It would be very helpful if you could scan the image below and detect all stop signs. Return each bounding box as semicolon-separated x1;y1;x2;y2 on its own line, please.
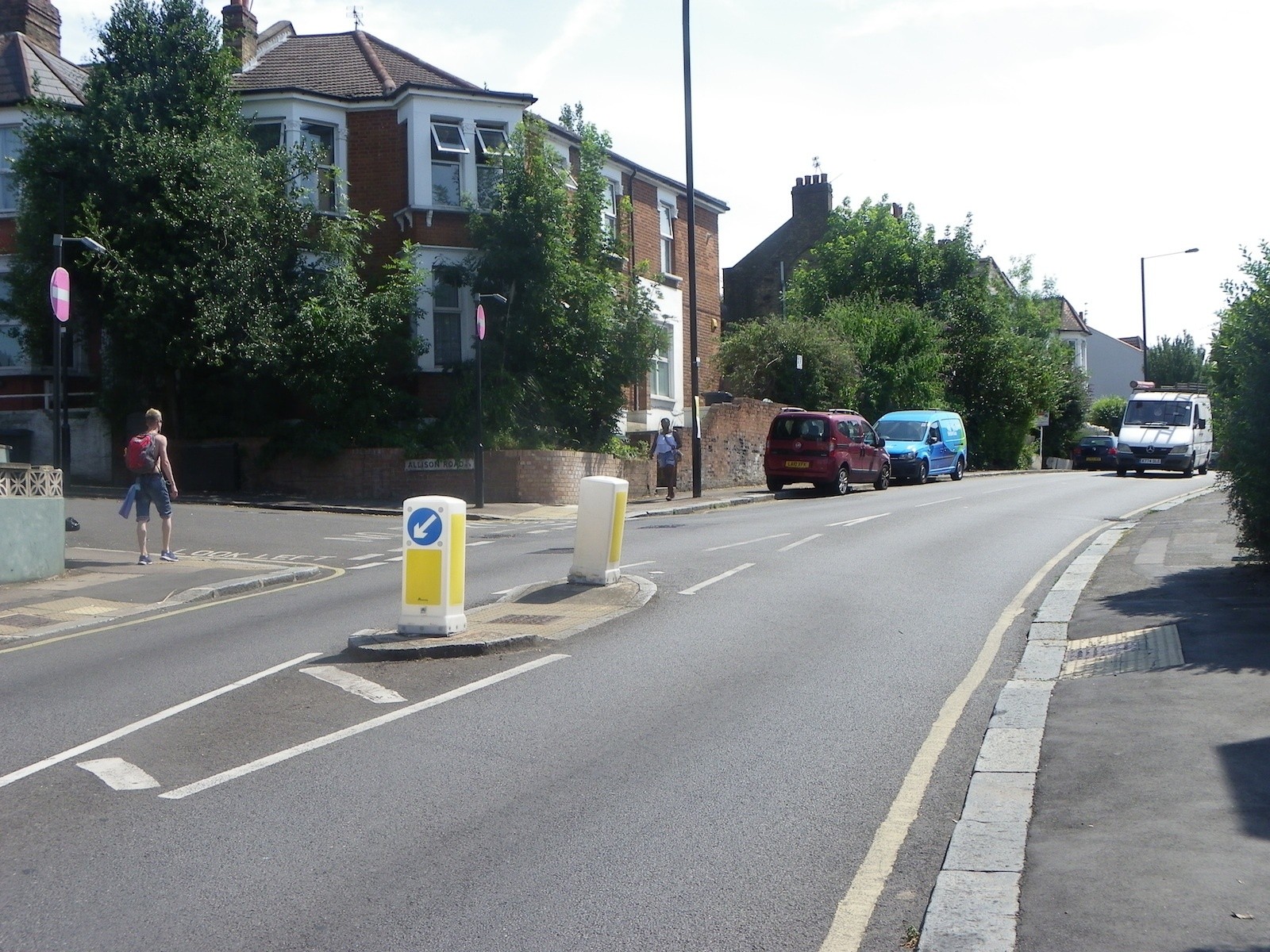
475;305;486;341
51;267;70;323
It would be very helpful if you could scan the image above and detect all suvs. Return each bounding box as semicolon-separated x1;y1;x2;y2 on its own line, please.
764;409;893;496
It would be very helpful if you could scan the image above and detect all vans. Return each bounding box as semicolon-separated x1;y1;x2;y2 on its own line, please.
863;409;968;485
1114;384;1212;478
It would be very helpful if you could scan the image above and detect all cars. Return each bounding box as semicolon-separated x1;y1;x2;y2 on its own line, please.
1074;436;1119;472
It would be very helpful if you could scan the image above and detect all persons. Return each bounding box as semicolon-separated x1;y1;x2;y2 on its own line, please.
132;409;179;565
650;417;683;501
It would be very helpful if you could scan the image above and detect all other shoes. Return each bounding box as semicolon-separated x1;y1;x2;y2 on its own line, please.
675;450;684;459
666;496;672;501
159;550;179;562
138;555;153;565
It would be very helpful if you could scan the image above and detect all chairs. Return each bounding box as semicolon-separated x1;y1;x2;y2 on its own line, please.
807;425;820;441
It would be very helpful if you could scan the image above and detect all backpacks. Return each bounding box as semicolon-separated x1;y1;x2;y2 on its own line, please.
125;433;161;475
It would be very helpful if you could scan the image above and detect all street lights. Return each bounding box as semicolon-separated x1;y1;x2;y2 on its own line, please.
51;233;108;460
1139;248;1200;383
472;291;508;510
